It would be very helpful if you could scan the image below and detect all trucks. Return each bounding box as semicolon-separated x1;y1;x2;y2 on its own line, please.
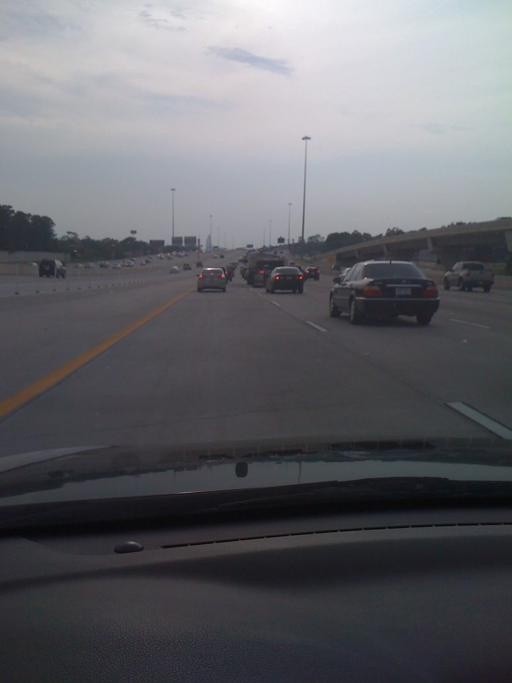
249;254;282;285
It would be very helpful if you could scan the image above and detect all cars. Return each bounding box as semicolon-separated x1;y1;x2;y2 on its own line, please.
39;258;66;279
266;267;304;294
169;260;203;273
196;267;228;292
305;266;320;280
443;261;495;292
329;260;440;326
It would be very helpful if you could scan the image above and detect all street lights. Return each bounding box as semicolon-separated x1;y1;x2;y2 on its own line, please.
170;188;176;246
301;135;311;240
268;217;272;247
288;201;294;249
